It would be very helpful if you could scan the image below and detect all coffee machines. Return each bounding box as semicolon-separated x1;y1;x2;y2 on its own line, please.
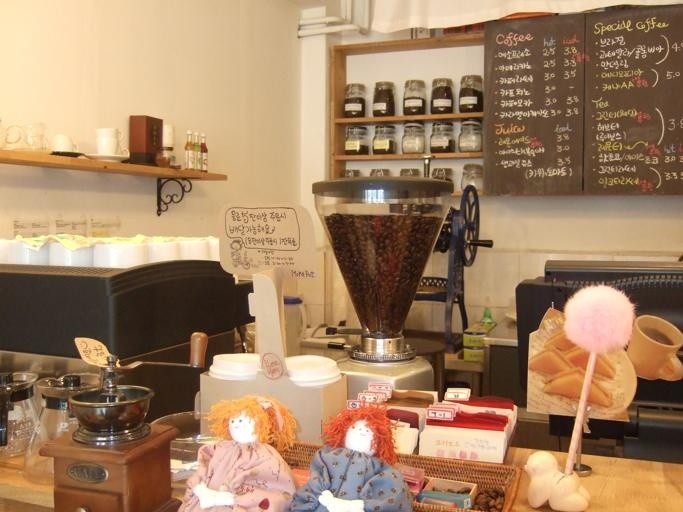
312;177;454;400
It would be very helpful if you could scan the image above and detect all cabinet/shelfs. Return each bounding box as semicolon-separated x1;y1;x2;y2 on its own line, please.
331;31;483;196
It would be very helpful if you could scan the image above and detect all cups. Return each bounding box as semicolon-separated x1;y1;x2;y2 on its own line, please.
627;315;683;382
96;128;125;155
284;355;341;388
209;353;261;380
52;134;79;153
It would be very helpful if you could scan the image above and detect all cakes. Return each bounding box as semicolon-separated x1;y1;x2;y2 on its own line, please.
528;330;615;407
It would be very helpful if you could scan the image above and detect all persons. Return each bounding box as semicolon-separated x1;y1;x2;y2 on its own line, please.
176;393;297;512
290;407;414;512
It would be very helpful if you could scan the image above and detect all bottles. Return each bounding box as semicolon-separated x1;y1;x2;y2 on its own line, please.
184;131;208;172
25;371;100;477
343;75;483;118
341;164;484;192
0;370;42;458
344;118;482;155
154;146;175;166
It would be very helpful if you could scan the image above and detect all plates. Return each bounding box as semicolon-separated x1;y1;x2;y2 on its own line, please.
527;324;638;421
49;151;85;158
84;154;129;163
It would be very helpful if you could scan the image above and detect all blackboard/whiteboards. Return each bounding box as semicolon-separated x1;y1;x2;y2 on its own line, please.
583;4;683;196
482;13;585;196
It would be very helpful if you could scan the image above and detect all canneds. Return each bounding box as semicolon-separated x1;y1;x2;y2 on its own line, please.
430;122;455;152
458;119;482;152
401;122;425;154
373;126;395;154
344;127;368;155
